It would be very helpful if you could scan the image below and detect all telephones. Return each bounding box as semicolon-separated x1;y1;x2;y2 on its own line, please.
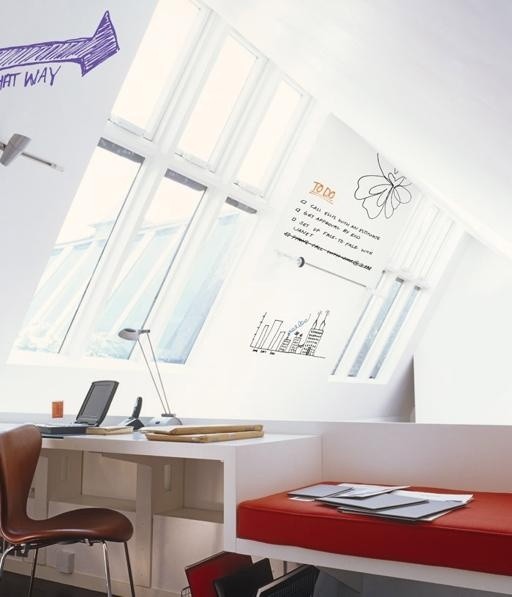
120;397;145;429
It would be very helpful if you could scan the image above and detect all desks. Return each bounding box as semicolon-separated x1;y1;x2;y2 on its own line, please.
237;479;510;596
1;422;323;596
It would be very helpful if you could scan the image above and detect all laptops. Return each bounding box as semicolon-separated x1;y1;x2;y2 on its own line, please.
33;380;118;435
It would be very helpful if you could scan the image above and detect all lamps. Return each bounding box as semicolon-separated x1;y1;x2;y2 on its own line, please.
118;328;182;428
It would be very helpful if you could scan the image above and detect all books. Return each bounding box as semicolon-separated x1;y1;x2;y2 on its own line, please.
286;478;477;525
85;425;134;435
212;558;273;597
184;551;253;597
254;564;320;597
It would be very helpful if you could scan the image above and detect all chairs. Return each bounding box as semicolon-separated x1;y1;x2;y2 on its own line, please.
1;424;134;597
